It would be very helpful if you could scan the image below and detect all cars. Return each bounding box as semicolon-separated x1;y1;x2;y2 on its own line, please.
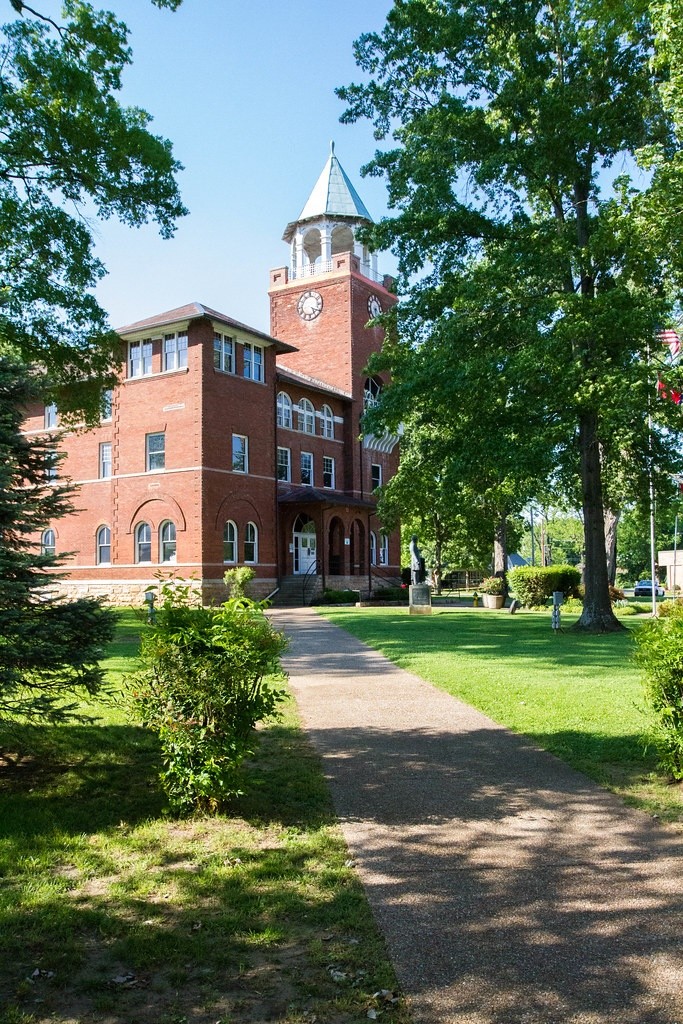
634;580;664;597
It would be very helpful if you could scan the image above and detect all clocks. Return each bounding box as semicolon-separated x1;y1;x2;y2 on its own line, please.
367;294;382;319
296;290;323;321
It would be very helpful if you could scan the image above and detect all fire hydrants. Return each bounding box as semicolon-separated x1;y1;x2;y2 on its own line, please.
472;591;478;607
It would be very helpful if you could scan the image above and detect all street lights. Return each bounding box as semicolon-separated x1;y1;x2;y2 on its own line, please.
530;506;539;566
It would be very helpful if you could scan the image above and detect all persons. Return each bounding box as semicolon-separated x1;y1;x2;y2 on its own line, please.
408;535;422;584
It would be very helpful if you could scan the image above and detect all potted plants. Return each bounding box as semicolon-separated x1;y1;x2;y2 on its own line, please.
478;576;508;609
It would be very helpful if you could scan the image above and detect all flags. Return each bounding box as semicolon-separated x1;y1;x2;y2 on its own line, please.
650;326;682;404
